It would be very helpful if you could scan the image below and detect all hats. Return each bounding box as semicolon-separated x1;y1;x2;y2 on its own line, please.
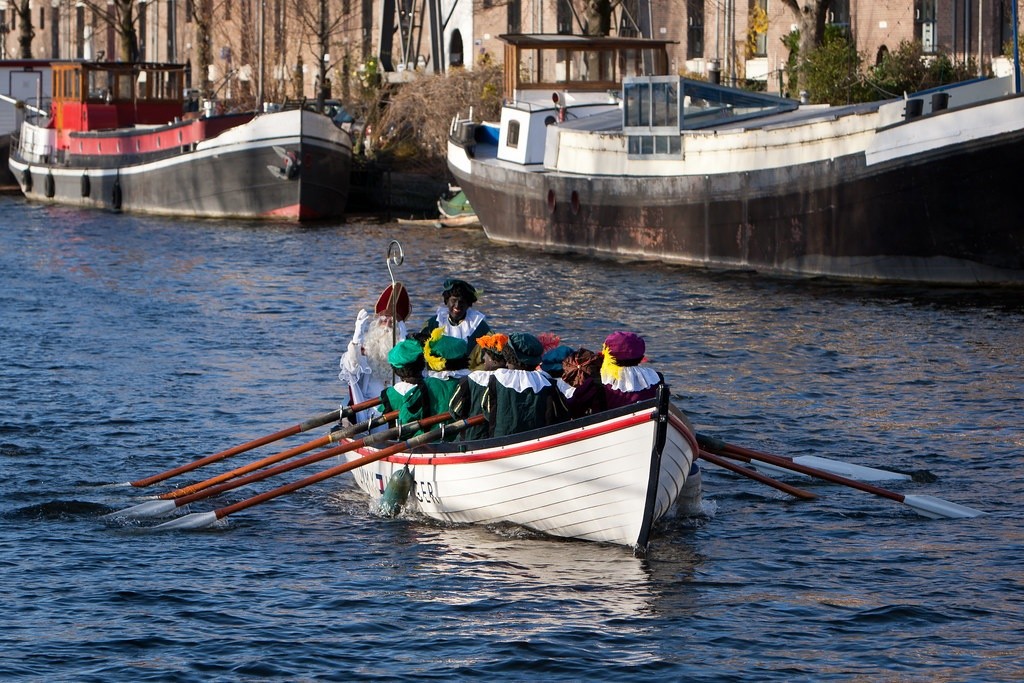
476;332;544;366
442;279;484;304
387;340;423;369
602;331;647;379
538;331;604;386
423;327;467;372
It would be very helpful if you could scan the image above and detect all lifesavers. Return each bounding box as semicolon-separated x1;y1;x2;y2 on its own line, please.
44;174;55;196
20;169;32;192
80;175;90;197
111;186;122;209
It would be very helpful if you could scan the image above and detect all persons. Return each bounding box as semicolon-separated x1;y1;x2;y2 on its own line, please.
340;278;664;443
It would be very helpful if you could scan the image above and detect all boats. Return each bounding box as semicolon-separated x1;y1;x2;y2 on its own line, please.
8;55;354;227
329;372;703;558
441;0;1024;292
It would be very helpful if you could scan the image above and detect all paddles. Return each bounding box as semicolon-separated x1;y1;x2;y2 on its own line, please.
101;394;487;534
695;431;987;520
